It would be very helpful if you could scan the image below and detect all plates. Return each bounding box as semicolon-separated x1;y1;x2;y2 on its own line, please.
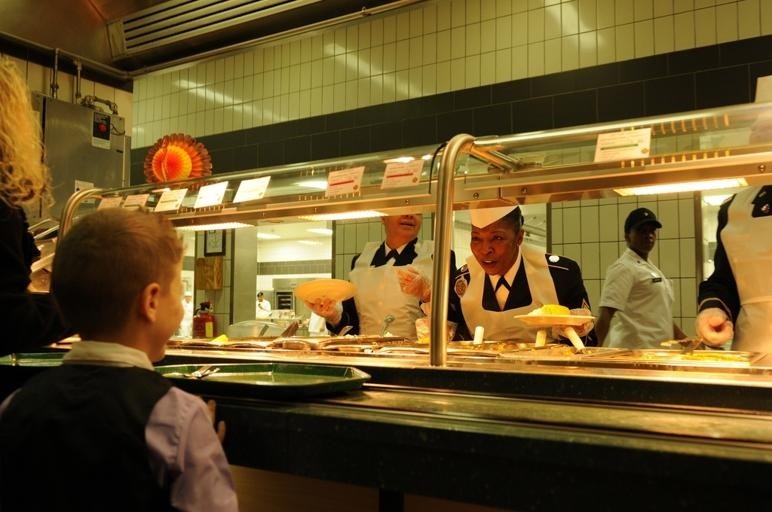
293;279;359;303
515;315;596;328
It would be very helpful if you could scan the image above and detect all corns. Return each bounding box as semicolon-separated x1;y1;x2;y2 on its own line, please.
541;305;570;316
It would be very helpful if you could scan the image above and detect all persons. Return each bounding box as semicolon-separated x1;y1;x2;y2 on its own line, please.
451;205;598;346
0;207;239;512
696;175;772;351
304;214;457;342
181;291;193;327
595;208;691;349
256;292;271;317
0;57;78;357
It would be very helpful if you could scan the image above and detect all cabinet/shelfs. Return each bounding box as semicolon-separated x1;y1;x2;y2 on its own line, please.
57;98;772;512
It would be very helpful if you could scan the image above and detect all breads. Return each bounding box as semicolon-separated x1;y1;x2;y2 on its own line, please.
570;307;591;316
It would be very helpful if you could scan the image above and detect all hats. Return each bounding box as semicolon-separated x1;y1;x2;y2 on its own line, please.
471;206;518;229
755;74;772;104
625;208;662;228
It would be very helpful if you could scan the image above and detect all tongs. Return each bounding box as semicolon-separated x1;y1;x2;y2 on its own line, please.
187;366;221;382
271;320;299;337
